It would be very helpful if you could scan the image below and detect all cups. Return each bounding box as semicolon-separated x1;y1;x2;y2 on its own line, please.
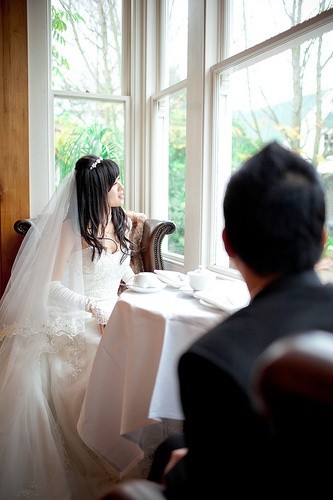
128;272;156;287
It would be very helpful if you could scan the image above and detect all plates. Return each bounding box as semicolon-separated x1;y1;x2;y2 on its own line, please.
179;285;195;296
199;299;223;310
126;282;167;293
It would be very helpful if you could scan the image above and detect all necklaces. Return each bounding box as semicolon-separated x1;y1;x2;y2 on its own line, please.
98;213;112;225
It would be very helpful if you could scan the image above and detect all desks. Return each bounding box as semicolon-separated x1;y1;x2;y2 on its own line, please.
77;286;251;477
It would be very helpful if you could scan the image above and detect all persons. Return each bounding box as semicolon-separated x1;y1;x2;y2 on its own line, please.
145;141;333;500
0;154;137;500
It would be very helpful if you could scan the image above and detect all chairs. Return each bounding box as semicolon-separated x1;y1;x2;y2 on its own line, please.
13;212;176;295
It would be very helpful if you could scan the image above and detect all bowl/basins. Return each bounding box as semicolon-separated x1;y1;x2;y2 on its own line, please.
187;266;218;290
